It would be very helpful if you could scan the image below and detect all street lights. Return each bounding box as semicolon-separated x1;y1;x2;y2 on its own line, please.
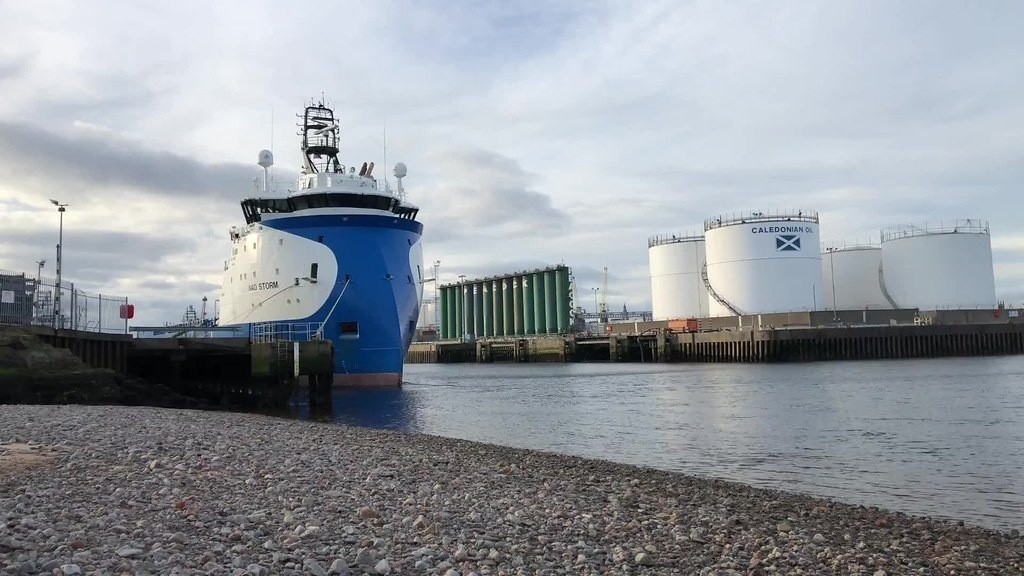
459;274;467;343
49;197;70;329
35;259;46;326
591;288;600;337
826;247;840;326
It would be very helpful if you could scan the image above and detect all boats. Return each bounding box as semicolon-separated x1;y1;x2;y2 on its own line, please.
137;91;435;390
